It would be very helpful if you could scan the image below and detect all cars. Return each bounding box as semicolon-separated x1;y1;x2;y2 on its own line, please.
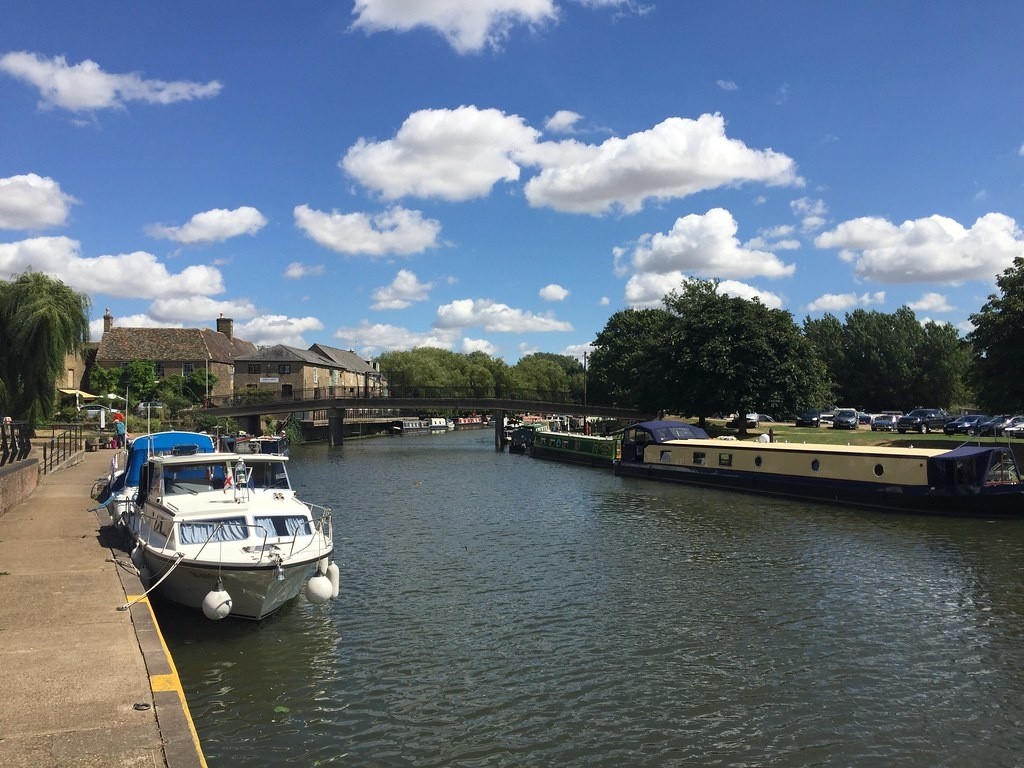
795;411;821;427
943;414;991;436
833;407;861;430
979;415;1024;437
1001;416;1024;438
55;404;120;421
734;410;760;428
133;401;167;416
870;415;898;432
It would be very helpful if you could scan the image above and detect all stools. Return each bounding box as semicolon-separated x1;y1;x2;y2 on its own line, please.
90;442;105;451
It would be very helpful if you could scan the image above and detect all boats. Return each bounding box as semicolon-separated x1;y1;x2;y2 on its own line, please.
508;414;570;454
534;431;622;468
101;387;334;622
615;420;1024;520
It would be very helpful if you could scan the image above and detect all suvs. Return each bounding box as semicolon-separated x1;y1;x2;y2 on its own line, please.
897;409;955;435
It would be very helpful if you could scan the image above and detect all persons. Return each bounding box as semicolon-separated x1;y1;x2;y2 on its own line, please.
114;419;126;448
